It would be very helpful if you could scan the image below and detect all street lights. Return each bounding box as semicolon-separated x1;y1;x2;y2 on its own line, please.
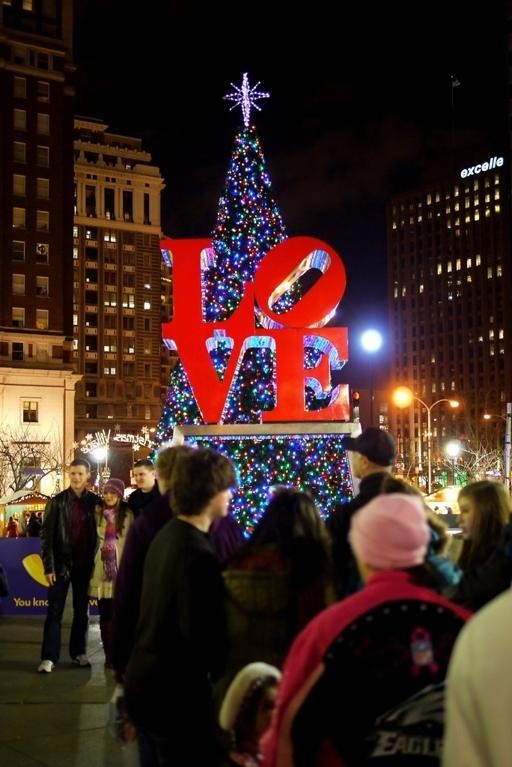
483;410;511;496
394;386;461;497
446;441;482;482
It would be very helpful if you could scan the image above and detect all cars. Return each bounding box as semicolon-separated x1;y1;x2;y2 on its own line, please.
422;484;463;531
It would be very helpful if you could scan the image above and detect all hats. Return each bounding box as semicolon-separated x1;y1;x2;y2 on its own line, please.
105;479;125;496
341;427;397;466
348;493;429;568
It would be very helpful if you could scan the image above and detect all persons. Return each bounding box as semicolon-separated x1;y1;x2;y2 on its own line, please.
104;423;511;767
84;477;135;669
34;457;105;674
6;510;43;537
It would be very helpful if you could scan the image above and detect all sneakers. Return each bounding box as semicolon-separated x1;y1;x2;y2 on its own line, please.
74;655;92;668
39;660;54;673
